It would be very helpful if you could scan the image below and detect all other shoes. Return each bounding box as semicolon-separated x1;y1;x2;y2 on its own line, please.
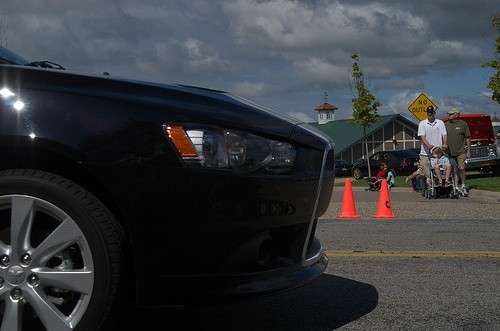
445;180;451;184
438;181;442;184
461;187;469;197
454;188;460;196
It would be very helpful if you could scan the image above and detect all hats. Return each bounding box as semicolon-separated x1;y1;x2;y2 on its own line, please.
447;109;460;115
380;162;385;167
426;106;434;112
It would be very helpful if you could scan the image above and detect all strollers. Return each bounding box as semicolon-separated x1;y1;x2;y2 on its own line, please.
424;145;460;200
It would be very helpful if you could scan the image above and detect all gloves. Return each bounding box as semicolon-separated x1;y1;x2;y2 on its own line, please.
405;177;409;183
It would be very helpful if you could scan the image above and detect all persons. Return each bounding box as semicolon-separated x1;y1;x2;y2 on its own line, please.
405;158;423;192
365;162;396;191
417;105;447;177
443;108;471;197
430;147;451;184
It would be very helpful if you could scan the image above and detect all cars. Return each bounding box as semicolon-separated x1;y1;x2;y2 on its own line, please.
352;149;421;180
403;148;422;155
335;159;352;177
0;43;336;331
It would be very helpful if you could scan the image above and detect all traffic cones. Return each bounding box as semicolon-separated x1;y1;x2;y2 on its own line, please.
372;178;397;218
336;179;362;218
